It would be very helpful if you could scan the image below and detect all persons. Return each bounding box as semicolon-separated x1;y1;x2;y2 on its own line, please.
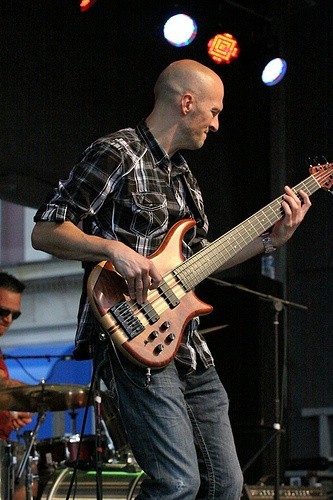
31;59;313;500
0;270;97;500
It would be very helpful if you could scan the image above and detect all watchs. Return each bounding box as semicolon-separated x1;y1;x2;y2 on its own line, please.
262;232;276;254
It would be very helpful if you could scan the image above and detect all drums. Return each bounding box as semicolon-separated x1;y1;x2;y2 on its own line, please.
45;432;115;469
35;434;69;467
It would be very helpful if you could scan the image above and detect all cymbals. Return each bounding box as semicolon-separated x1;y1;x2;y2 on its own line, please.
1;384;94;410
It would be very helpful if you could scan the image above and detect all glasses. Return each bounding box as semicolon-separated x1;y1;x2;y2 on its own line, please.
0;305;21;320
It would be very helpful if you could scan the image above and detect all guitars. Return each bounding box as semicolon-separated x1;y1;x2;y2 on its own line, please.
88;163;333;368
